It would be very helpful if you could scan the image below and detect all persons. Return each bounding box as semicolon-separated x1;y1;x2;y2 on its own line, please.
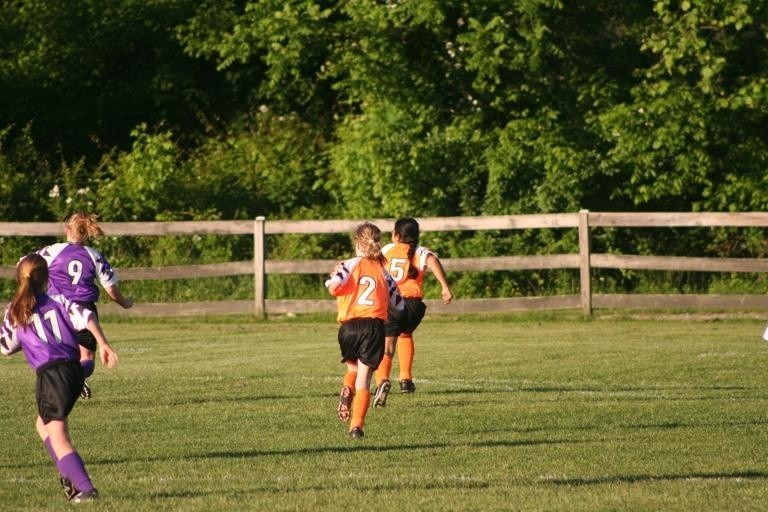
16;212;136;399
324;220;407;440
0;253;120;504
371;218;454;411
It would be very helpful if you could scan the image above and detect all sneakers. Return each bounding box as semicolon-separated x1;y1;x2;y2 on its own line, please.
337;385;353;423
372;379;391;408
400;379;415;392
80;381;91;400
60;477;98;503
349;427;364;441
